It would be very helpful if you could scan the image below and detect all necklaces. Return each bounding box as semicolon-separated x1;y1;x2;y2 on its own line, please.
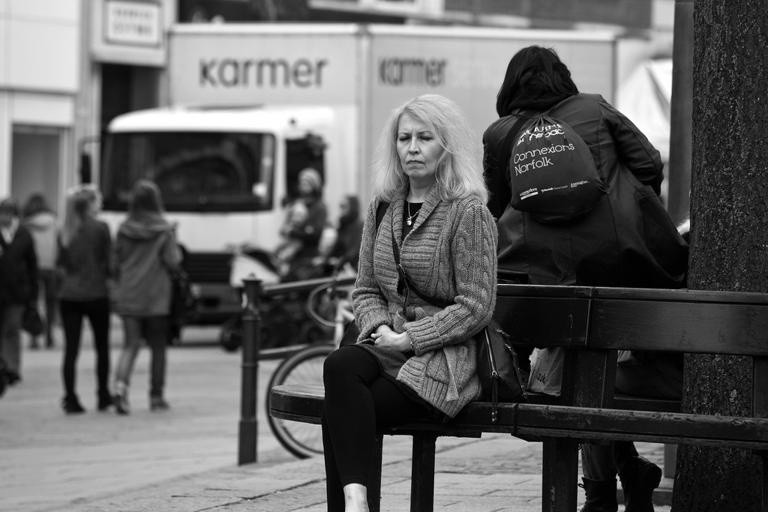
406;201;419;227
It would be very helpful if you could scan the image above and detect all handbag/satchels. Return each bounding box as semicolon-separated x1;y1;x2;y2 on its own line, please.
478;320;524;402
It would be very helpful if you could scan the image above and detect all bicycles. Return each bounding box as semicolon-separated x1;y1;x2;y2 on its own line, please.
267;255;358;458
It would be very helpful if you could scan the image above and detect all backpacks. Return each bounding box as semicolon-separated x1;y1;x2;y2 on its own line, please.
508;111;604;215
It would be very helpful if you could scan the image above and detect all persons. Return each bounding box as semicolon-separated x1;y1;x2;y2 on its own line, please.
577;219;691;512
322;93;527;512
107;179;178;414
54;183;115;413
319;195;365;279
281;168;327;282
0;198;39;386
483;46;690;391
21;195;63;350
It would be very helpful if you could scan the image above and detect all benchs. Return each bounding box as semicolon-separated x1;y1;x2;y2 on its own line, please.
267;282;768;511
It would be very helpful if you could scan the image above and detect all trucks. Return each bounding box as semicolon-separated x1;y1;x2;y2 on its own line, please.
76;25;620;321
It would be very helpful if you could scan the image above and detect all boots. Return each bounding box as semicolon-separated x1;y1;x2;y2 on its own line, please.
579;457;662;512
